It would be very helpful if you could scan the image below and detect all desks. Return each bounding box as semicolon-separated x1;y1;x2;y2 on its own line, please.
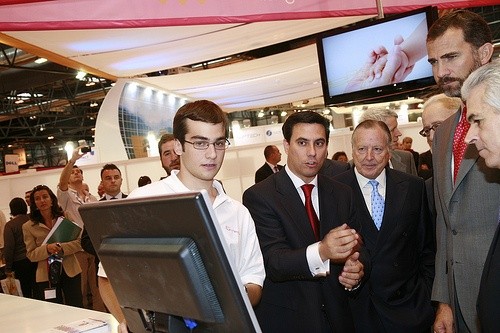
0;288;121;333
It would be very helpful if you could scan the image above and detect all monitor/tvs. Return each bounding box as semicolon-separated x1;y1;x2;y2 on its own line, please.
316;6;438;108
76;192;263;333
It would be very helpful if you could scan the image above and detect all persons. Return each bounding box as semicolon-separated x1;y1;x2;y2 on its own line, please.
0;146;129;314
138;176;151;187
344;17;429;93
241;93;461;333
425;10;500;333
96;100;266;333
158;134;181;177
460;60;499;333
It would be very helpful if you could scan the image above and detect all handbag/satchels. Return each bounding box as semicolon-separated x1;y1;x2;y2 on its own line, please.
47;257;62;289
1;275;24;297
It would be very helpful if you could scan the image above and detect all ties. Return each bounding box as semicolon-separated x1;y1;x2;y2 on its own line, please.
370;181;384;231
301;183;320;242
275;167;278;172
453;106;470;185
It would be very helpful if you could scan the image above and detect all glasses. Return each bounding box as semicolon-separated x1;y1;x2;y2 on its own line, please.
419;123;441;137
184;138;230;150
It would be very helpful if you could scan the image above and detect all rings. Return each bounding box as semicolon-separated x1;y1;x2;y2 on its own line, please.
355;279;359;284
56;243;61;247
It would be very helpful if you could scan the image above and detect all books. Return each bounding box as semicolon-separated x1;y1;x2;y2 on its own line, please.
41;216;83;246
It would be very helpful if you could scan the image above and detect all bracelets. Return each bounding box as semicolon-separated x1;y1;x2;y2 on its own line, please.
68;160;75;165
